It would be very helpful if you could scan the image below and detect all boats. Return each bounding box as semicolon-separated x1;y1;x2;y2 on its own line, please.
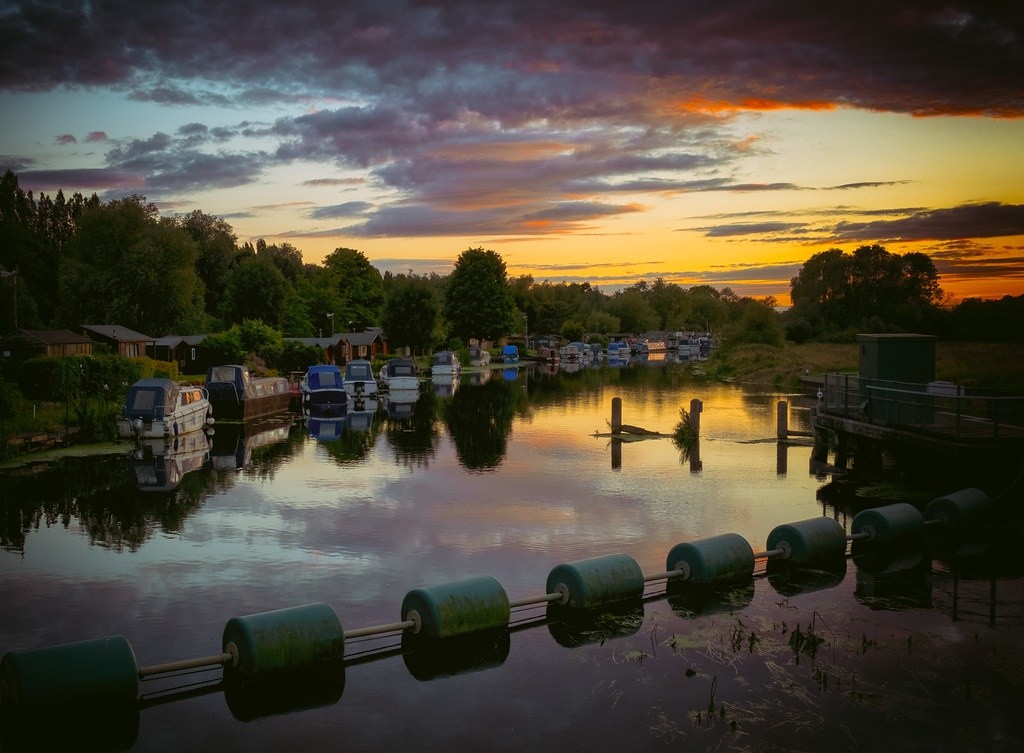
380;359;421;390
301;364;353;405
536;338;709;360
204;365;292;423
430;351;461;375
470;347;492;366
344;360;378;398
501;346;520;363
115;378;211;439
